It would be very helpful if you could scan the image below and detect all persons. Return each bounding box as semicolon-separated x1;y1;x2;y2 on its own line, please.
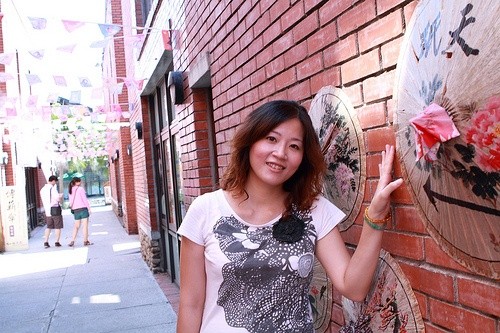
40;175;63;248
68;177;91;247
176;100;403;333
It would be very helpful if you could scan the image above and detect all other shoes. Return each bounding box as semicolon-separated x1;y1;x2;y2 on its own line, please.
69;241;74;247
84;241;93;246
44;242;50;247
55;241;61;246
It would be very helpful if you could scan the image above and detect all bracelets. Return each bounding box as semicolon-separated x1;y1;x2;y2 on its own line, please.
363;215;384;230
365;207;392;223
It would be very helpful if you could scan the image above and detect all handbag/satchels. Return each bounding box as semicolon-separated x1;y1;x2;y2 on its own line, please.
51;207;61;216
